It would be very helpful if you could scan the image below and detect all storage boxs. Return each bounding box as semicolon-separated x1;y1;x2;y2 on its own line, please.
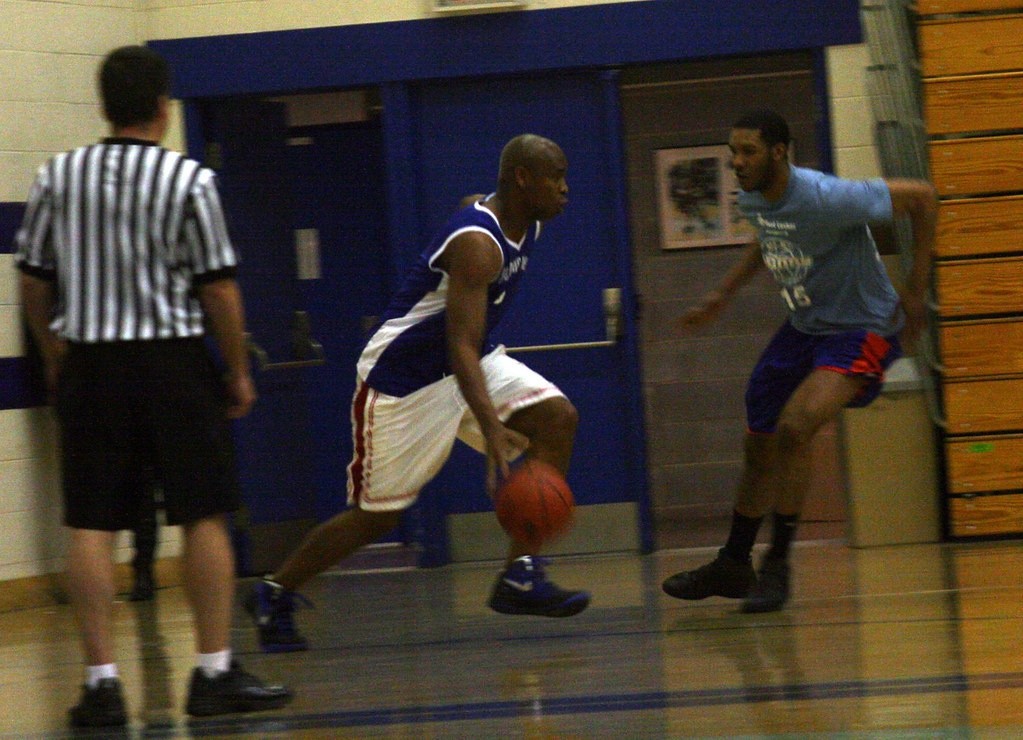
909;0;1023;539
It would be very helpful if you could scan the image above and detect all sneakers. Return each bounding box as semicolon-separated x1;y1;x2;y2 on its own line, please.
187;660;294;716
67;679;127;726
662;556;752;600
490;554;589;617
240;575;315;652
742;562;788;611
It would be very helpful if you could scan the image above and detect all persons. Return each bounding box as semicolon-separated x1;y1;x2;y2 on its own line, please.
11;44;294;731
241;134;591;654
659;111;940;615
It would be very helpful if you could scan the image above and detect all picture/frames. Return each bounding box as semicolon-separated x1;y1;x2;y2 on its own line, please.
651;138;797;254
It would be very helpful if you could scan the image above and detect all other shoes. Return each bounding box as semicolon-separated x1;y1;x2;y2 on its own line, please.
129;585;156;601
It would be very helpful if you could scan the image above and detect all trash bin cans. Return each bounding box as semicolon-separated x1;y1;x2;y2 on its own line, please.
840;357;947;548
797;417;849;522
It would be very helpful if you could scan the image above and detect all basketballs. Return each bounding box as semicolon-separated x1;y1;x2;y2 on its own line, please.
495;458;577;547
